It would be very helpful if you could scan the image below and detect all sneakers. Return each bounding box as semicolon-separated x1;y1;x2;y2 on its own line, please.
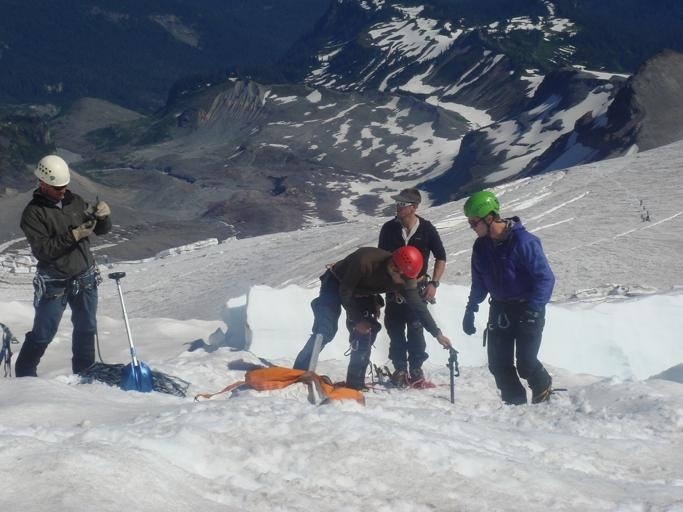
533;377;553;404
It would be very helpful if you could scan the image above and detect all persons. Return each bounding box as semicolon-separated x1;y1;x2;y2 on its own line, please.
15;154;113;378
291;187;453;392
462;190;556;407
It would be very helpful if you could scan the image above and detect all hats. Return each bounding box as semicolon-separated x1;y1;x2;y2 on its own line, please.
391;187;421;204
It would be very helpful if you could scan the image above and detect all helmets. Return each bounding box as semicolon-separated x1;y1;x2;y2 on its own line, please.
34;155;70;187
464;191;501;217
393;245;424;280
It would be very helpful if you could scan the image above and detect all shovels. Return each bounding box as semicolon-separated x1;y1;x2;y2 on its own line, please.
108;272;154;390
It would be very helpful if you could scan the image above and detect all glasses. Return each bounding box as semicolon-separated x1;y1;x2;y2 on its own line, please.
395;201;411;207
470;219;483;228
